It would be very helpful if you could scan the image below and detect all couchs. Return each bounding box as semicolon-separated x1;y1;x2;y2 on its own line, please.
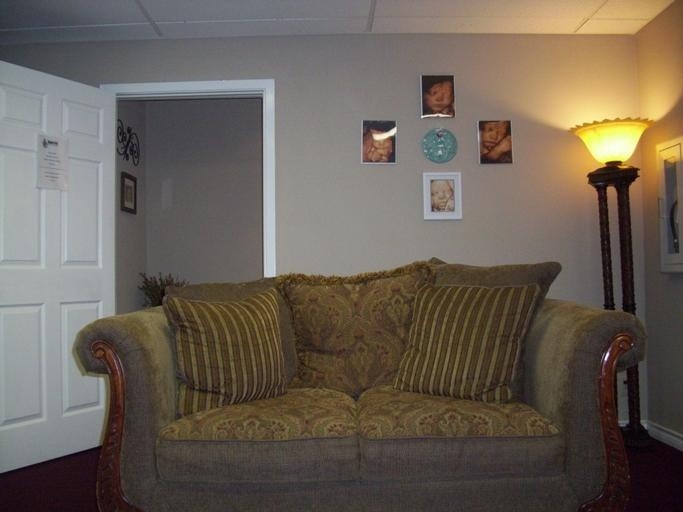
70;295;649;512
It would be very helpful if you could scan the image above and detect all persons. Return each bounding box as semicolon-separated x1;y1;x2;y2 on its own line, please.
363;128;393;164
429;180;454;213
422;79;454;117
478;120;512;165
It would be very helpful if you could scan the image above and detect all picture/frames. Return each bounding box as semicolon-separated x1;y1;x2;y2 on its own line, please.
120;171;137;214
423;172;462;222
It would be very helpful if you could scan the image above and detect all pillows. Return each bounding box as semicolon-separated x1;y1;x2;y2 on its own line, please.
393;277;540;401
162;288;286;421
278;261;434;397
165;276;299;388
427;258;562;297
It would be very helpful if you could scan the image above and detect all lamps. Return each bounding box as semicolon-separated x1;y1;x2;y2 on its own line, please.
572;118;653;448
